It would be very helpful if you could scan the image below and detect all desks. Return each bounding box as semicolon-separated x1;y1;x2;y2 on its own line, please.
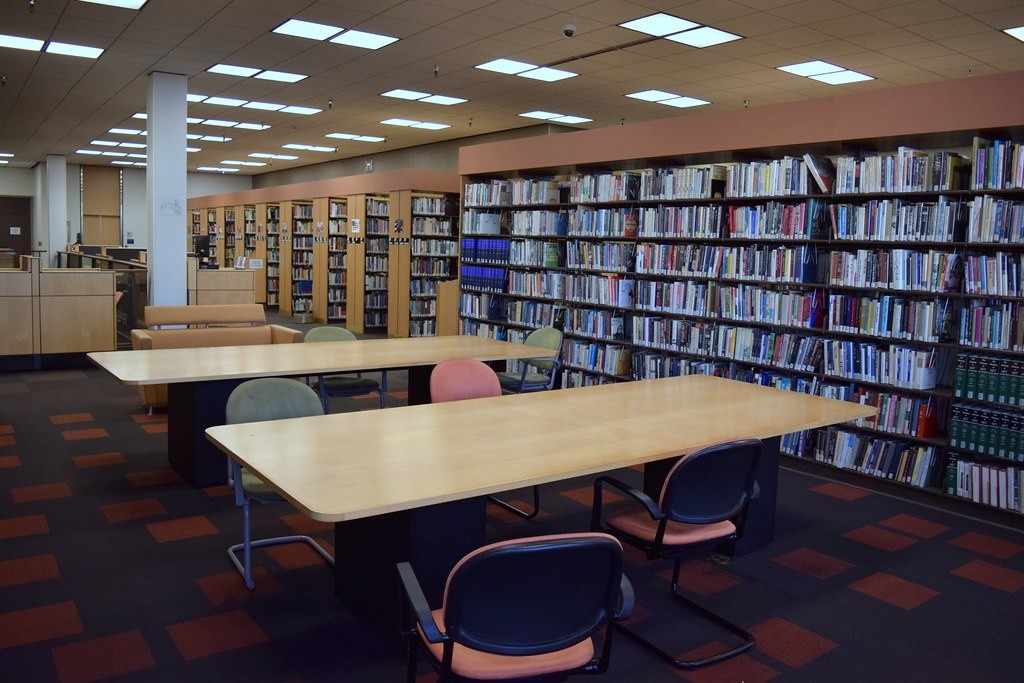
203;372;878;636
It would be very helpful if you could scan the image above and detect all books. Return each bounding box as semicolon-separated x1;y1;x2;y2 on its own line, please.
193;196;458;337
459;137;1024;515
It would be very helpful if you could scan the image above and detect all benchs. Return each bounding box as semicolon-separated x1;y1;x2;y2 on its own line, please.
128;303;305;417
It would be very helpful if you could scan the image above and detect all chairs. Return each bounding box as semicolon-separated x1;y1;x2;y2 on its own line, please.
394;532;624;683
429;358;541;519
86;333;558;490
582;436;767;668
495;327;563;397
225;378;335;585
303;325;388;416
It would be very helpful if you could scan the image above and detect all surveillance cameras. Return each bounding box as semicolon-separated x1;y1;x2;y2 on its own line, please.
561;24;576;38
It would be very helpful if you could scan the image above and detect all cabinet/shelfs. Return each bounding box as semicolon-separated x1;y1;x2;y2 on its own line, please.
184;70;1024;514
0;256;118;368
